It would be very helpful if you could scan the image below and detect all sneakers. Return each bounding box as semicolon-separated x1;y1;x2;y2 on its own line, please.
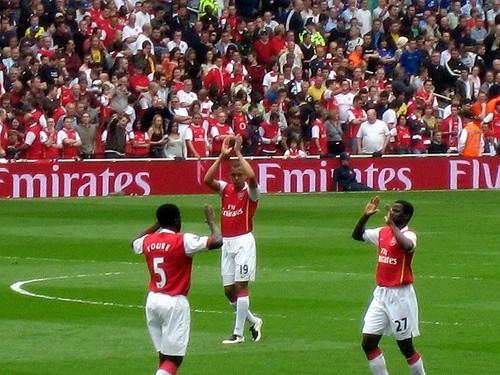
221;335;245;344
249;318;263;342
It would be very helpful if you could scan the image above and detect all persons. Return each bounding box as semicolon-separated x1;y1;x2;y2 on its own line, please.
0;0;500;193
352;196;427;375
204;134;263;344
130;203;223;375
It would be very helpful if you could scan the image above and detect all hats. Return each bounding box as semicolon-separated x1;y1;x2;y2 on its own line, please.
339;151;351;161
